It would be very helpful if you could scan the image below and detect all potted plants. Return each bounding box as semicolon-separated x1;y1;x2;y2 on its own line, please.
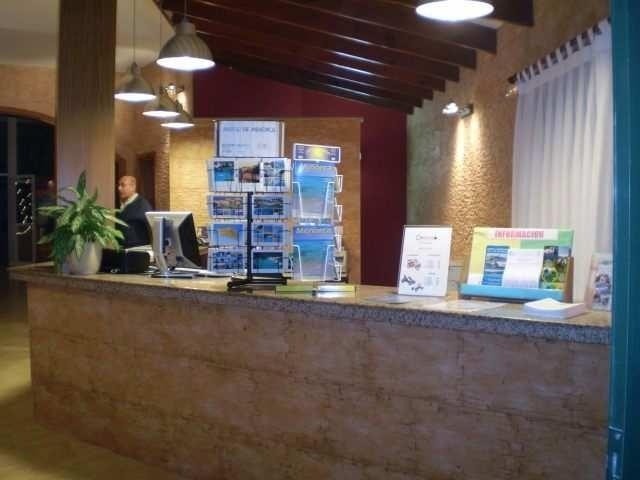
34;166;132;276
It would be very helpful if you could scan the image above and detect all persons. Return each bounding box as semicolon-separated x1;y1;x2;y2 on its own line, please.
116;175;153;249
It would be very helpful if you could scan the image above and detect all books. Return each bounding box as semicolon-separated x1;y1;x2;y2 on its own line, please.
208;158;337;281
523;297;589;320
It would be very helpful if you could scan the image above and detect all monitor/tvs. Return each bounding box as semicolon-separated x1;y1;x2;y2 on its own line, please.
144;211;203;278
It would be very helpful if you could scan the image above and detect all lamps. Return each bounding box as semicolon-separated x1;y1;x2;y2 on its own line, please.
113;1;215;129
